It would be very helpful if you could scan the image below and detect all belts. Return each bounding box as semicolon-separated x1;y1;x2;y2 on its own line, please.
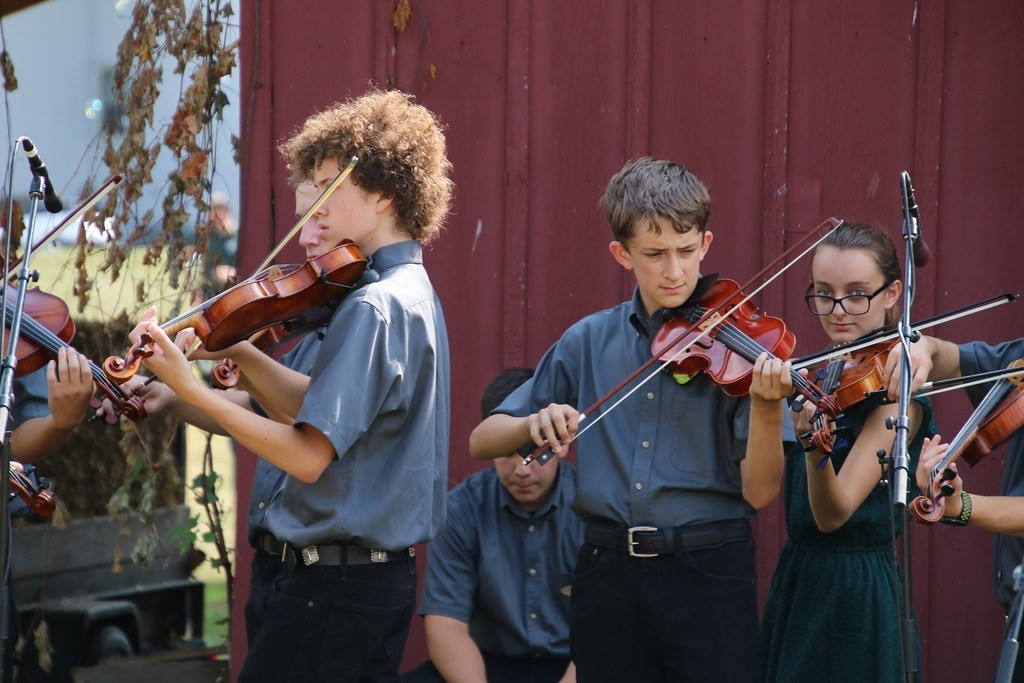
261;532;284;555
281;542;415;571
586;519;752;557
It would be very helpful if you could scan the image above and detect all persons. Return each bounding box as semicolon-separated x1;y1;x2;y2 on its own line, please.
883;333;1024;683
468;156;808;683
758;220;938;683
128;87;452;683
0;175;584;683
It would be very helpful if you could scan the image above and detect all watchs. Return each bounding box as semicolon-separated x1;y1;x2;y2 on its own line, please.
940;490;972;526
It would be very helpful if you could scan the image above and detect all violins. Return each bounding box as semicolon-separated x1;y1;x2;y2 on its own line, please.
8;463;57;518
908;357;1024;526
0;277;147;423
208;299;344;391
800;338;901;455
651;277;842;421
101;238;368;386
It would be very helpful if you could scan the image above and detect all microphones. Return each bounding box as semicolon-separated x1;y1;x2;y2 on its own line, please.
20;136;63;213
899;170;932;265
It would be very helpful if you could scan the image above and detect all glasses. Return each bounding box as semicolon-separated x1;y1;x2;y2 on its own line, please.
805;277;898;315
